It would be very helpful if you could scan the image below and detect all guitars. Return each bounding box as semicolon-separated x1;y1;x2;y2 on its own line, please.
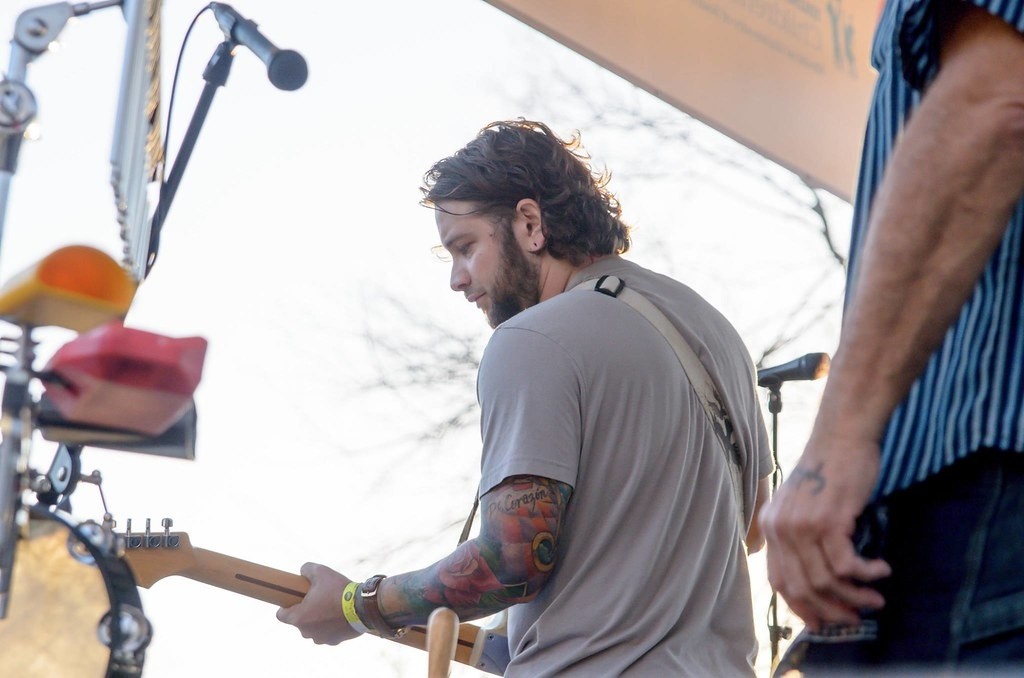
92;509;510;674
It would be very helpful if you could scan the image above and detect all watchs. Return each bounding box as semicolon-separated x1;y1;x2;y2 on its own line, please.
361;575;412;639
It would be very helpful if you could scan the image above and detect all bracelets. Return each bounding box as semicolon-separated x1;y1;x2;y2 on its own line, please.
341;581;373;633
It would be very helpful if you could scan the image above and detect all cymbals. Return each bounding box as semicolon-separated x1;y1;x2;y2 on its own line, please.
2;503;147;678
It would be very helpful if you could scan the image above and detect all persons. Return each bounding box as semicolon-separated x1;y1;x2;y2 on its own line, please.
276;119;774;678
758;0;1024;677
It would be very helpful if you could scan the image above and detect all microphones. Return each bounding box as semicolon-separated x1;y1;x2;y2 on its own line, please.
754;351;835;386
210;0;309;92
